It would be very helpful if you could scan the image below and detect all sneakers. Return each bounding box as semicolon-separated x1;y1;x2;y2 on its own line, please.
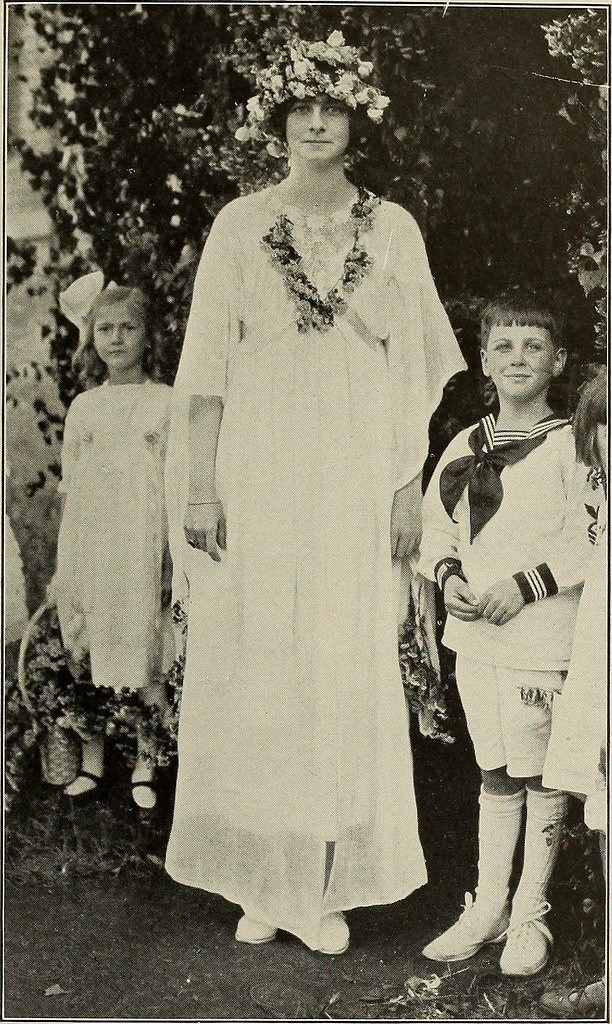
490;912;555;980
418;889;510;965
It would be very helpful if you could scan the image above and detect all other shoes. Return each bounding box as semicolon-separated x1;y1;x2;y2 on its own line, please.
313;912;352;957
234;913;277;947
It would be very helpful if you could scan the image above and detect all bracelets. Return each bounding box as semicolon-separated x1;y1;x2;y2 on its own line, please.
186;499;222;506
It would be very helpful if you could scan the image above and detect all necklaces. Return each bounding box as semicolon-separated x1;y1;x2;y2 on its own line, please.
258;180;377;333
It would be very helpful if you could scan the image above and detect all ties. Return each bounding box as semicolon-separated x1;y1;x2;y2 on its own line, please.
439;418;549;546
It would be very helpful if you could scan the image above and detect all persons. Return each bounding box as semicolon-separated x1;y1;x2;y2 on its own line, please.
399;295;594;976
534;360;609;1019
46;290;173;828
163;28;470;958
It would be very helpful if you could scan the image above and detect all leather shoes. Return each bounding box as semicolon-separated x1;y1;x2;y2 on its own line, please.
60;771;109;811
126;777;161;825
540;980;606;1020
